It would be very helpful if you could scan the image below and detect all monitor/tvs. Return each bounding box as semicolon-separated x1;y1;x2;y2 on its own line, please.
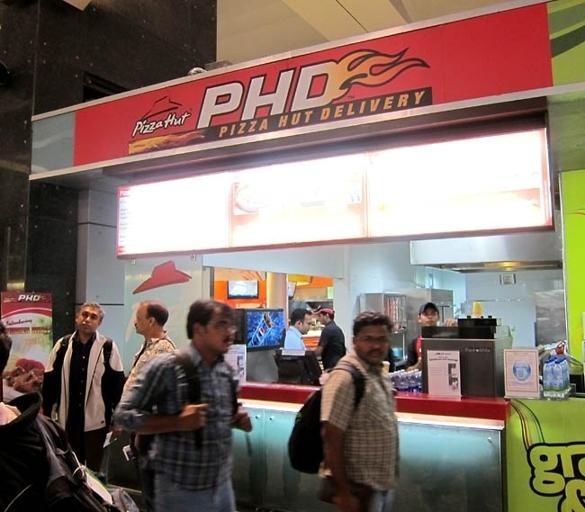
272;347;323;383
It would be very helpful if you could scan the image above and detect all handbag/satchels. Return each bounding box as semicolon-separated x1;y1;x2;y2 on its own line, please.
37;415;141;512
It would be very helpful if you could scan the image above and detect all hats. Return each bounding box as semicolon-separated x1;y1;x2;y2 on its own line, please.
313;309;335;315
419;302;439;315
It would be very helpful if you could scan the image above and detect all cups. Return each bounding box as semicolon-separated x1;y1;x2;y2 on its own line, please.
249;314;273;347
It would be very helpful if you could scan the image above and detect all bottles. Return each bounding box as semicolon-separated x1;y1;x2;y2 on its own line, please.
389;370;423;394
542;356;569;395
568;391;569;394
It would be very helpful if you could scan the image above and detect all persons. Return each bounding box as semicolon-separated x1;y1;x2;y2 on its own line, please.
404;302;458;372
320;309;401;512
42;305;125;473
105;301;176;511
282;308;313;354
1;321;83;512
312;308;346;371
113;297;254;512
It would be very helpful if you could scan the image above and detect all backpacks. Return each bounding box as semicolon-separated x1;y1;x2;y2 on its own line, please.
288;364;363;476
130;351;238;468
